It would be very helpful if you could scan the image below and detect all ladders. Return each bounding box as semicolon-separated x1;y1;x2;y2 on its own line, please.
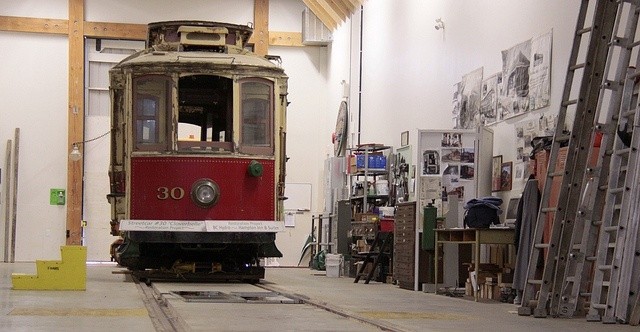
518;0;618;317
557;0;640;322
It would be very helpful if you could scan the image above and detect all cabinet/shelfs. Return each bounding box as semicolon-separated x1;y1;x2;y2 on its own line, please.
349;146;393;255
534;146;599;263
393;201;429;291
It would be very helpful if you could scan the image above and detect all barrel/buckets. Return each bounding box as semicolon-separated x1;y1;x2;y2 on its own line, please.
324;253;342;278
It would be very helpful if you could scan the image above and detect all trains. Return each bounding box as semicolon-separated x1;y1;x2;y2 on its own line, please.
105;20;292;285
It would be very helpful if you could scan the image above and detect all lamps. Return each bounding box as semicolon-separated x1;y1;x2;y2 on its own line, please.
69;143;82;162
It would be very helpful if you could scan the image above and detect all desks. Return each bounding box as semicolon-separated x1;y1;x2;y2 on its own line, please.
433;227;516;302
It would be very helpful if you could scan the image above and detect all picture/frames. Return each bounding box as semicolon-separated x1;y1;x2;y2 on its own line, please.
500;162;512;191
492;155;503;192
441;147;475;163
459;163;475;182
400;131;409;146
441;132;462;149
420;149;441;177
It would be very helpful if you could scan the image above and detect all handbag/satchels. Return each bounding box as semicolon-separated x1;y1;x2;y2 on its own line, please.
464;205;500;228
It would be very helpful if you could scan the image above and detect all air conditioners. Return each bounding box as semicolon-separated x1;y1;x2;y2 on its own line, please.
302;8;334;46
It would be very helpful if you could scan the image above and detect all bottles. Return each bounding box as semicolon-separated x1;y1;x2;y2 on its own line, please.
465;278;472;297
370;185;374;194
